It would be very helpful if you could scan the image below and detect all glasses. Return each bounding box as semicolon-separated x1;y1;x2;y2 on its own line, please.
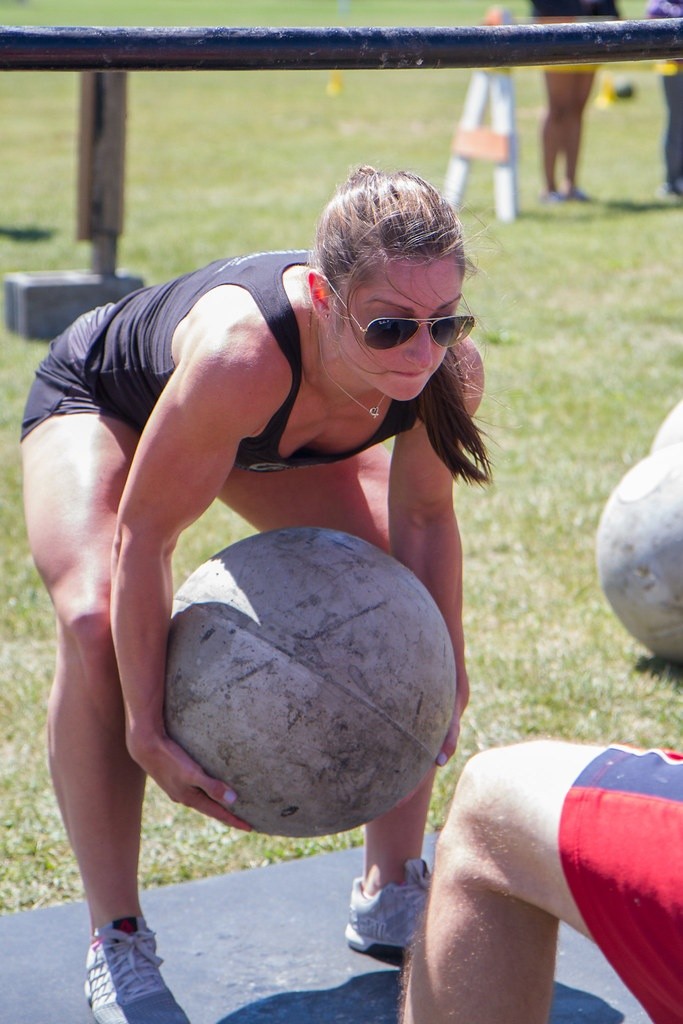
325;279;475;350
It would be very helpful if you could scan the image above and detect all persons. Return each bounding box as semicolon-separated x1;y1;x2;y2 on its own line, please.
17;163;504;1022
396;396;683;1023
643;0;683;197
530;0;623;206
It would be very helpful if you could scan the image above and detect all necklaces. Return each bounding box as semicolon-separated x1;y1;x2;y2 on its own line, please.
317;319;386;420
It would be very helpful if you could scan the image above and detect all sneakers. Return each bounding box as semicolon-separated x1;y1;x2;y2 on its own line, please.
84;916;191;1024
344;859;431;957
